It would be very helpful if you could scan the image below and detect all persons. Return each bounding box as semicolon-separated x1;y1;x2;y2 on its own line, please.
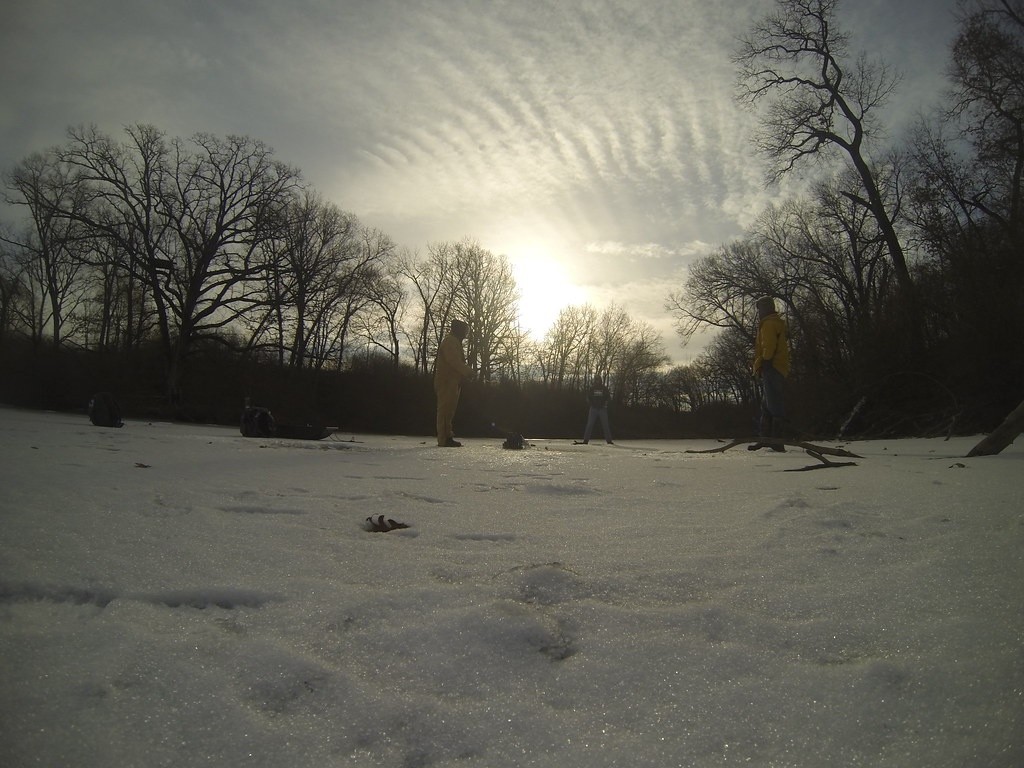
747;297;790;452
435;320;473;447
583;373;614;444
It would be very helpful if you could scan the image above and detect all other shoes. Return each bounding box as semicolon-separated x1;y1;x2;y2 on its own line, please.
439;438;461;447
747;439;770;450
583;440;588;444
771;439;784;452
607;440;613;444
